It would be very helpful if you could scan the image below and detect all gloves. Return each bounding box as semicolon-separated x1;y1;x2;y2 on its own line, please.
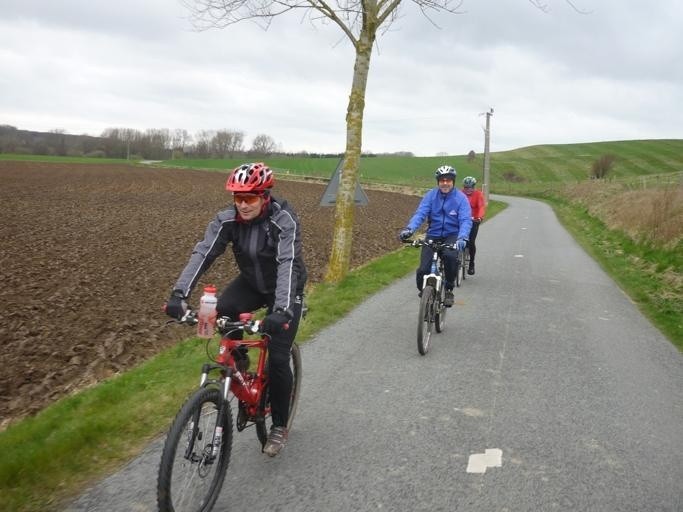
164;292;191;320
456;240;467;252
399;230;413;239
260;310;294;334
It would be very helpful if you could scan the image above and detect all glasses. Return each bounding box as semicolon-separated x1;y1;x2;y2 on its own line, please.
439;178;452;183
232;192;263;205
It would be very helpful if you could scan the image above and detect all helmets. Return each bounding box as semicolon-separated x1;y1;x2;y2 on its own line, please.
435;166;456;179
226;162;274;194
463;176;476;187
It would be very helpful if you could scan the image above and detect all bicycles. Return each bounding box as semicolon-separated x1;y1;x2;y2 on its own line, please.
155;303;303;512
461;216;480;281
401;239;458;355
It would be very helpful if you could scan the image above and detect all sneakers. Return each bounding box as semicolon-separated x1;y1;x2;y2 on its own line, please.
220;354;249;377
443;291;454;308
468;263;474;275
263;425;288;454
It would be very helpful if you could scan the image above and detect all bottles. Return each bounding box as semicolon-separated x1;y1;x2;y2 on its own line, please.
196;284;218;340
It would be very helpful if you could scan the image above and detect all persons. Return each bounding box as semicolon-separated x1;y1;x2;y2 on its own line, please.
459;176;486;275
399;165;473;308
166;162;307;458
